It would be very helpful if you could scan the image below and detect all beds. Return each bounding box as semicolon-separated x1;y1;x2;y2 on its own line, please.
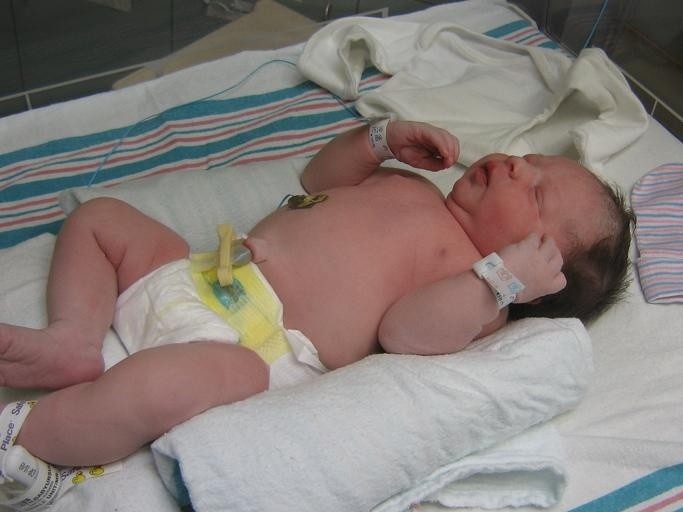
0;2;683;512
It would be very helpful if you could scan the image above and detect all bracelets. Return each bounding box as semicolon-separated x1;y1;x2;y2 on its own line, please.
369;116;398;164
472;248;527;317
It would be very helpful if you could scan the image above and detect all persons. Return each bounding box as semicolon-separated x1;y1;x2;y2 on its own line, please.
0;120;637;470
